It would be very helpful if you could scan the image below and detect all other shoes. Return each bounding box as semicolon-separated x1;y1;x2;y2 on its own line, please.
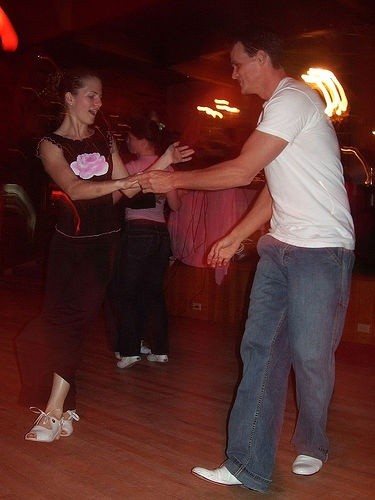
117;355;141;369
147;354;168;363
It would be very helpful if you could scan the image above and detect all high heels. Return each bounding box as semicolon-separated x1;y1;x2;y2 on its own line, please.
24;410;60;442
59;408;78;437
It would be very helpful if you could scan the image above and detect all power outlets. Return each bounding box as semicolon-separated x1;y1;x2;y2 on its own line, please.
357;324;371;334
192;302;202;311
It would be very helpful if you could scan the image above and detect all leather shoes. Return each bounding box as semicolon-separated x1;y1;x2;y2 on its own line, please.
292;443;328;475
192;465;257;491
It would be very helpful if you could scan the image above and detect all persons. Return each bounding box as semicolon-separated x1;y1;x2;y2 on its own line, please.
136;23;357;494
97;116;182;370
23;64;195;447
